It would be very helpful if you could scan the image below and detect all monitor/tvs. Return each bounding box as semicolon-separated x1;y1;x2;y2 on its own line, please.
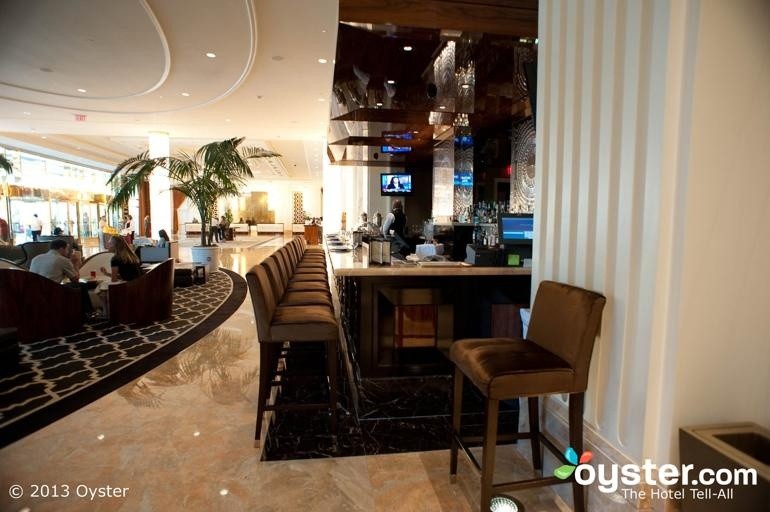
381;131;415;153
455;135;472;146
498;213;533;244
454;169;474;188
380;173;414;197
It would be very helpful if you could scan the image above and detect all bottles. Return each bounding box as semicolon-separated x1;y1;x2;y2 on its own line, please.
453;114;473;145
454;59;474;98
471;224;501;249
410;223;424;234
451;199;532;224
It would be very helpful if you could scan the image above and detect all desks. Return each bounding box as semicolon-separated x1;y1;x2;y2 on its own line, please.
304;225;317;244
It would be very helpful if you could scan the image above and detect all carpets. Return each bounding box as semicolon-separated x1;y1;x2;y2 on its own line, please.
1;261;248;449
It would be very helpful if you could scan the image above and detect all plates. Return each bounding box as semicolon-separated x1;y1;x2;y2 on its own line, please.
327;234;353;251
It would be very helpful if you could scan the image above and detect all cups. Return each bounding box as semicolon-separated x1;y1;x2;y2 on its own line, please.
90;271;96;279
152;239;158;243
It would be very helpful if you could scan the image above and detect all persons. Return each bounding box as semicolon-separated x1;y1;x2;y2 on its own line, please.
209;215;258;244
26;212;149;252
384;176;405;190
192;217;199;224
155;229;170;248
95;234;146;319
28;239;99;320
310;215;324;243
381;199;408;237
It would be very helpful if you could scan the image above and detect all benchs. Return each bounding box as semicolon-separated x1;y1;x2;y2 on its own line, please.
185;223;305;233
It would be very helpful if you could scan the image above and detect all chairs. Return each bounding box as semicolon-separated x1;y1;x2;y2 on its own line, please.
0;234;178;343
449;280;606;512
245;235;342;450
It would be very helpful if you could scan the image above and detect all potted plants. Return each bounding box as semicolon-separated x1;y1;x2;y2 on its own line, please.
103;137;278;270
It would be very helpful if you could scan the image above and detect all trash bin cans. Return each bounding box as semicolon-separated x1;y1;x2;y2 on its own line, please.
678;420;770;512
